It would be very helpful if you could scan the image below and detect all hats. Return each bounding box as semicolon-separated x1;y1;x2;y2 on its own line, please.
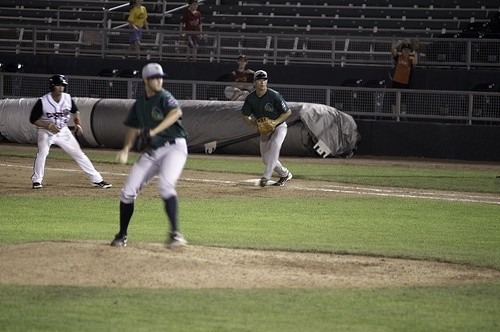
237;55;248;61
142;63;166;82
188;0;198;5
254;70;267;79
400;43;413;53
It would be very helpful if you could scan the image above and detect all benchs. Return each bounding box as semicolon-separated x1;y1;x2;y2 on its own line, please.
0;0;500;63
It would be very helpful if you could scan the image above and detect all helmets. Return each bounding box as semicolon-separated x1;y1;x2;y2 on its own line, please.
49;75;68;85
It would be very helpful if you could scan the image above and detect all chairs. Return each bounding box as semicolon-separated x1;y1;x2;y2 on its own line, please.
0;62;24;95
460;83;497;125
87;68;140;100
335;78;387;121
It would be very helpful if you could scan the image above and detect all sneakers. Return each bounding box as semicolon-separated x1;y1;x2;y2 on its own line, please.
165;233;186;248
275;172;293;186
260;177;267;188
32;183;43;188
111;235;127;247
91;182;112;188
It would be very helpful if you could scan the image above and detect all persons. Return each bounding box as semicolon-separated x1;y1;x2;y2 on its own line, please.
30;75;112;189
180;0;202;62
242;70;292;186
391;40;419;121
111;63;188;247
225;55;255;100
125;0;150;59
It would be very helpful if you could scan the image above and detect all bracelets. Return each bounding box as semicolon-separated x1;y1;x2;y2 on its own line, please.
74;118;80;125
48;123;52;130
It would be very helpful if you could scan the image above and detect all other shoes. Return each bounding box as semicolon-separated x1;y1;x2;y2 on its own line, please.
402;113;408;120
393;115;398;120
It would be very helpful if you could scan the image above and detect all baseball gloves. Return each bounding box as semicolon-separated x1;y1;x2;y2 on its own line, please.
135;128;153;154
255;117;276;132
